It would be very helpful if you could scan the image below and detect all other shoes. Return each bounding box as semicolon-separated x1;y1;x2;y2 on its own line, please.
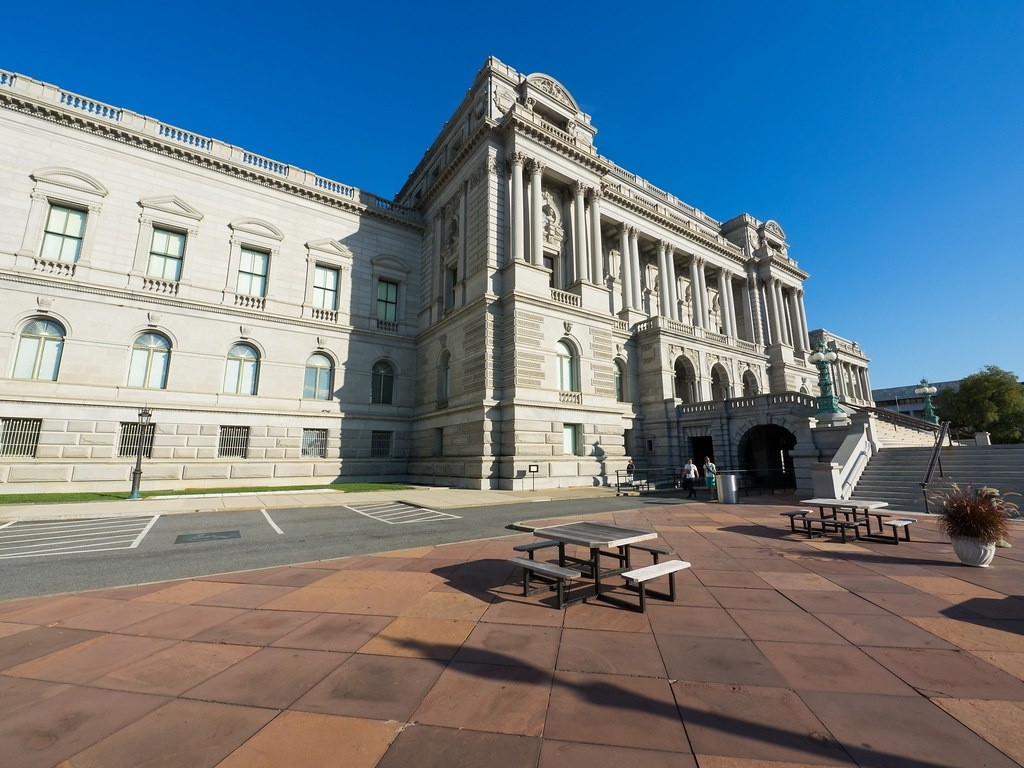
694;492;696;497
686;495;690;499
711;496;714;499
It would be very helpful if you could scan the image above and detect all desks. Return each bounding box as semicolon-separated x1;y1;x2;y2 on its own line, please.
534;520;658;598
799;498;888;539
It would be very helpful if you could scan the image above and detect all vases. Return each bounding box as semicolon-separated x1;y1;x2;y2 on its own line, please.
947;533;996;568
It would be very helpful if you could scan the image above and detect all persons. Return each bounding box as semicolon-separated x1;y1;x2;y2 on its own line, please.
627;460;635;485
566;120;577;137
682;458;699;499
703;456;716;500
524;96;536;111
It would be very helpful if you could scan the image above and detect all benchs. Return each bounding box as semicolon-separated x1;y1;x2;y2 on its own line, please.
616;544;676;571
781;510;814;533
883;518;918;545
621;560;690;613
507;556;581;610
836;510;891;531
513;542;570;580
794;516;859;544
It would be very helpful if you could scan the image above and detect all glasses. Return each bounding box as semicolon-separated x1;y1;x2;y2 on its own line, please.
706;460;710;461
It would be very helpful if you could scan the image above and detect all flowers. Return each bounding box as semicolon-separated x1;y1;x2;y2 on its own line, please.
924;476;1022;544
